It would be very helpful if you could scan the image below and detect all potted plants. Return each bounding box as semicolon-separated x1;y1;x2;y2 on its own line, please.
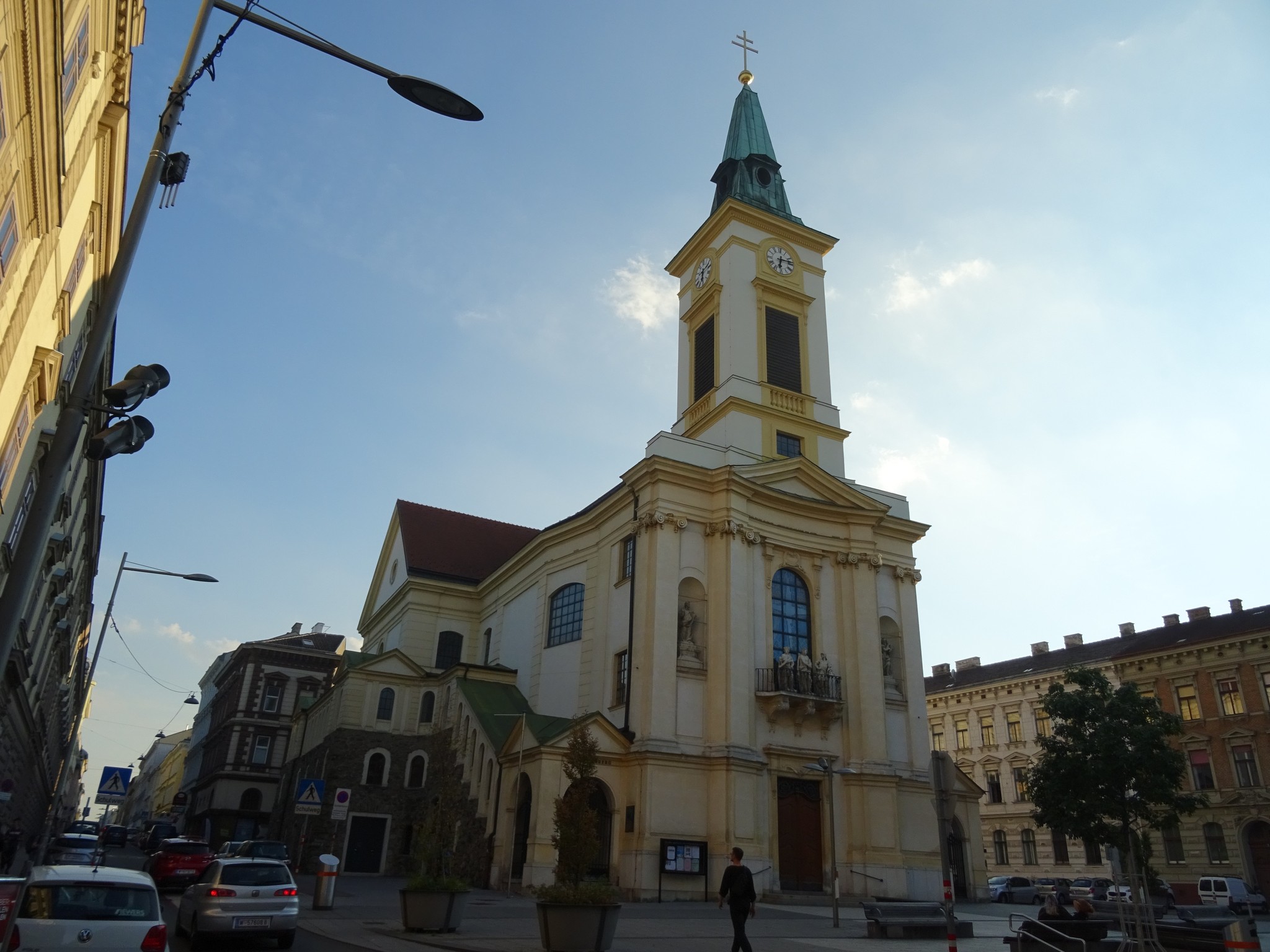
397;727;495;931
529;714;630;952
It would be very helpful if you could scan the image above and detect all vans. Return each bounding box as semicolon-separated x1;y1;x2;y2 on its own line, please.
1197;873;1267;915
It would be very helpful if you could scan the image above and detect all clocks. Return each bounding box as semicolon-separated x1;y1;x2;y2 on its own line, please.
695;258;711;288
767;246;794;275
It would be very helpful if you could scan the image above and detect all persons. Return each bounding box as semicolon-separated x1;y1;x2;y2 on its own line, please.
815;652;833;697
679;600;696;645
1037;894;1073;941
31;831;49;860
797;648;816;695
0;818;23;874
1073;899;1101;941
719;847;756;952
779;647;797;692
881;637;892;676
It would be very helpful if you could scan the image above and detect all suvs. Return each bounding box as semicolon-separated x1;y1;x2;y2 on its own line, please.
1105;875;1176;911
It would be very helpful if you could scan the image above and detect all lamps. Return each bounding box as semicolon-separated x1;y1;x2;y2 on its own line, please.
137;755;145;760
184;691;199;704
155;731;166;738
128;764;134;767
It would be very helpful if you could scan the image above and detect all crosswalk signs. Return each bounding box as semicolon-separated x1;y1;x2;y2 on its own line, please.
297;778;326;805
97;766;133;796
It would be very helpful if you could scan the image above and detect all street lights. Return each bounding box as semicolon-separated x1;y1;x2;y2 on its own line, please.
1;0;487;684
801;756;858;928
35;551;220;866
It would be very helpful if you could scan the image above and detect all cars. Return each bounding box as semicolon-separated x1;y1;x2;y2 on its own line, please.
69;820;178;851
8;864;168;952
987;876;1042;906
214;839;292;870
176;856;299;952
46;833;105;866
1033;877;1074;905
142;837;216;888
1068;877;1115;901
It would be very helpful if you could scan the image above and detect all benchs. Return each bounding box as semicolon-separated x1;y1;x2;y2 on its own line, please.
1003;900;1254;952
862;896;974;943
